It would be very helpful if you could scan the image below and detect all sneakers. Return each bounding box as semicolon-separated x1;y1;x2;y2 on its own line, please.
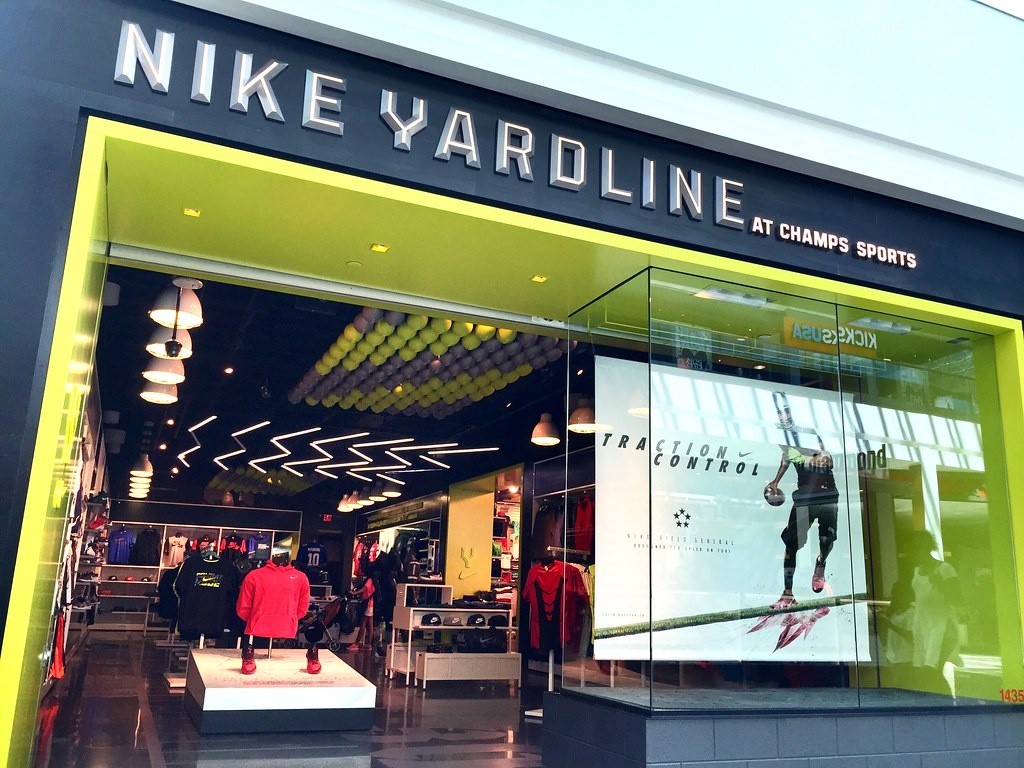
375;651;384;658
347;643;360;650
360;644;372;650
241;644;256;674
306;644;321;674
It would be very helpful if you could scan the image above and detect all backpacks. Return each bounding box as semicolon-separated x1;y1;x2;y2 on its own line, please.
332;593;360;635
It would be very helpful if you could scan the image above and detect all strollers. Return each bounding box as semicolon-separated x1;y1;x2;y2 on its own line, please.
283;588;356;655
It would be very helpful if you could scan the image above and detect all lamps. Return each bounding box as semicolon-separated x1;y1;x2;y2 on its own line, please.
568;399;595;434
138;276;203;406
529;413;562;447
336;473;402;512
127;454;153;499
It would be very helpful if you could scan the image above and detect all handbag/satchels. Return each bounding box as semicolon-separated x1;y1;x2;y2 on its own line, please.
354;599;368;627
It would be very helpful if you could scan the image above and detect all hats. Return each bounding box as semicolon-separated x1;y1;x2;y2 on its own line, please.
443;615;463;626
108;575;118;581
124;576;136;581
141;577;151;582
421;613;442;625
467;614;486;626
488;615;508;627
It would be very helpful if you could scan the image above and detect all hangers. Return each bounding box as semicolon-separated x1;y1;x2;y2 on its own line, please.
119;524;127;532
145;527;154;533
538;499;553;512
542;545;589;567
201;546;220;560
579;490;589;505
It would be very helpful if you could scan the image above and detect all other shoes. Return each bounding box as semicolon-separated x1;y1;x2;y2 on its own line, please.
68;536;109;609
420;572;442;582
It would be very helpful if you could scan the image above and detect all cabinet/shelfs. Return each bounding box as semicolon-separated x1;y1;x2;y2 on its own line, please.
69;504;107;630
383;583;523;690
510;560;519;579
85;519;300;632
492;516;506;578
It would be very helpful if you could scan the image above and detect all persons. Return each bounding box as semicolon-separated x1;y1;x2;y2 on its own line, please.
302;611;328;624
904;529;962;695
872;582;916;689
763;394;839;612
347;570;375;651
